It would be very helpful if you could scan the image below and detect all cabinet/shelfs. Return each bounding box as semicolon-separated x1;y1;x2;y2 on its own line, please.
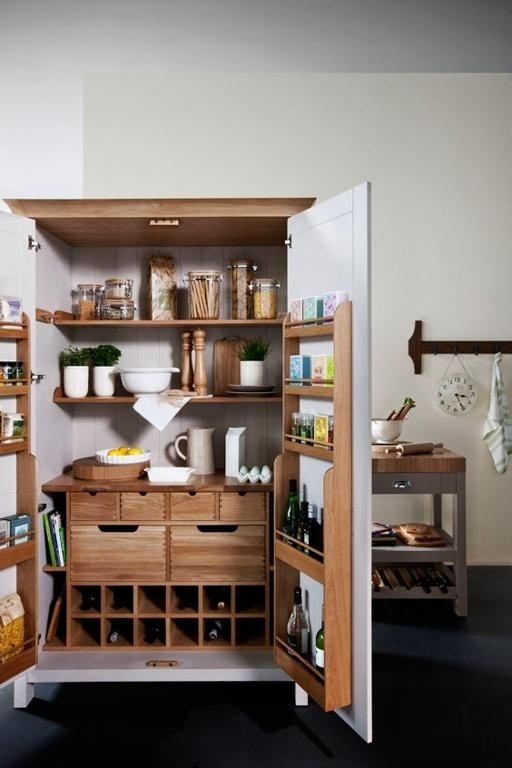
0;178;374;749
370;439;470;632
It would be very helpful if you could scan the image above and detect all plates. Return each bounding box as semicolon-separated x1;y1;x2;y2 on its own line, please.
225;384;280;397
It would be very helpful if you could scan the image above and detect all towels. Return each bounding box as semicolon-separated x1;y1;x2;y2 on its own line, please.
481;350;512;477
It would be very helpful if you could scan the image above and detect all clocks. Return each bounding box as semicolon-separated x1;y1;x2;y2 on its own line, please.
431;370;479;416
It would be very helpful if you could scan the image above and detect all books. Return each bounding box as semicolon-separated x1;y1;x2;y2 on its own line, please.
46;594;63;644
44;508;65;568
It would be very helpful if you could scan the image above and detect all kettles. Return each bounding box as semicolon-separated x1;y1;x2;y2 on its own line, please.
174;425;218;477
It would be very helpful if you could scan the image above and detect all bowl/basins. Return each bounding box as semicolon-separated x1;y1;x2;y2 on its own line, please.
370;418;403;445
113;367;181;398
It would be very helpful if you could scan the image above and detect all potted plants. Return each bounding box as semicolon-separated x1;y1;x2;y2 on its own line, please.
90;343;123;398
58;341;95;398
229;333;275;387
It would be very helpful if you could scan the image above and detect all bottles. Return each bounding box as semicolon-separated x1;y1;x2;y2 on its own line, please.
78;592;227;644
314;603;325;673
78;278;137;320
184;257;279;319
1;361;23;388
287;586;308;656
280;477;320;560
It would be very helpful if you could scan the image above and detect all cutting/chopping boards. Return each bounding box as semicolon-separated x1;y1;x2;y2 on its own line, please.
388;522;448;547
